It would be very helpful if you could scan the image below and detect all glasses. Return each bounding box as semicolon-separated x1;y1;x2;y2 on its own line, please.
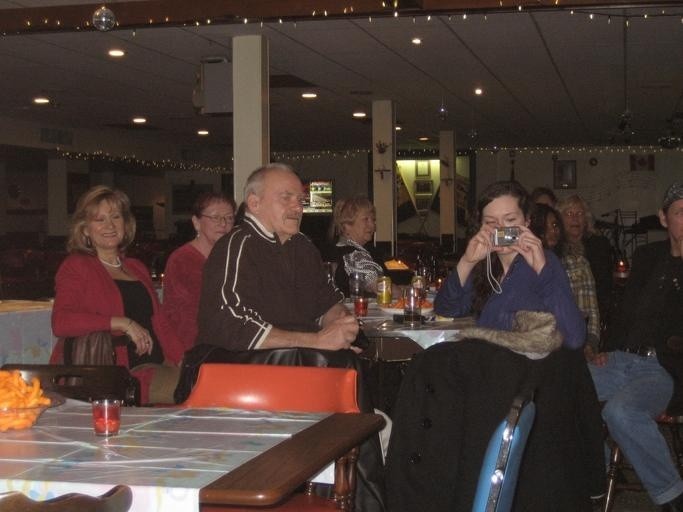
199;212;236;225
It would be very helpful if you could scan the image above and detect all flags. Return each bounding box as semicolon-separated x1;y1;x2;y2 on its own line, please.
430;172;473;228
393;167;416;226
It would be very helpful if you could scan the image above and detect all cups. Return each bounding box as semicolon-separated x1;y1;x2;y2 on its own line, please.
350;272;365;301
325;260;343;278
91;400;120;435
400;287;421;326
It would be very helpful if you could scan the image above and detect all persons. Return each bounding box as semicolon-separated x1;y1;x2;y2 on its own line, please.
430;182;588;354
159;192;238;364
49;188;185;408
198;162;370;355
526;184;618;362
581;178;683;511
328;194;389;298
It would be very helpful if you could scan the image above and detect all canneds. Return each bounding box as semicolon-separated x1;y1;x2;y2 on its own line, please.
375;276;392;304
411;276;425;298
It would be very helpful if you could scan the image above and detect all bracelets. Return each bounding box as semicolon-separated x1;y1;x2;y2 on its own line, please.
124;320;134;335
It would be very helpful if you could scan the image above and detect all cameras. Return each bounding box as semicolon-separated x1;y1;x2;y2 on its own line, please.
492;226;522;248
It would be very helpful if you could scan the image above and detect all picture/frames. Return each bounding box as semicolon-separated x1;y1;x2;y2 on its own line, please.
412;178;434;197
413;159;431;179
552;159;577;189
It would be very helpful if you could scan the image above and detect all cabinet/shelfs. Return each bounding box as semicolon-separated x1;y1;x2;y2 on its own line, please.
309;182;332;208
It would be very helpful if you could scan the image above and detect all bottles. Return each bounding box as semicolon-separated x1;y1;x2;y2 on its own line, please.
411;250;438;283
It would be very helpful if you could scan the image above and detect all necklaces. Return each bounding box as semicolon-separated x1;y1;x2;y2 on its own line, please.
97;253;122;269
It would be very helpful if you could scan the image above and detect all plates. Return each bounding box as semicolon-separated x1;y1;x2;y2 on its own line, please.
342;297;432;313
0;390;67;435
